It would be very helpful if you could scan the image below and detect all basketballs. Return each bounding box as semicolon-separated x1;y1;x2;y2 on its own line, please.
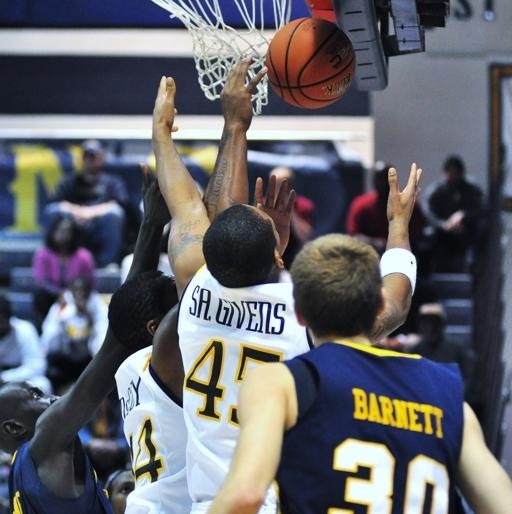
266;18;355;110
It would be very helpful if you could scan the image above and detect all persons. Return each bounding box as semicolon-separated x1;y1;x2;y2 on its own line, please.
1;56;512;513
